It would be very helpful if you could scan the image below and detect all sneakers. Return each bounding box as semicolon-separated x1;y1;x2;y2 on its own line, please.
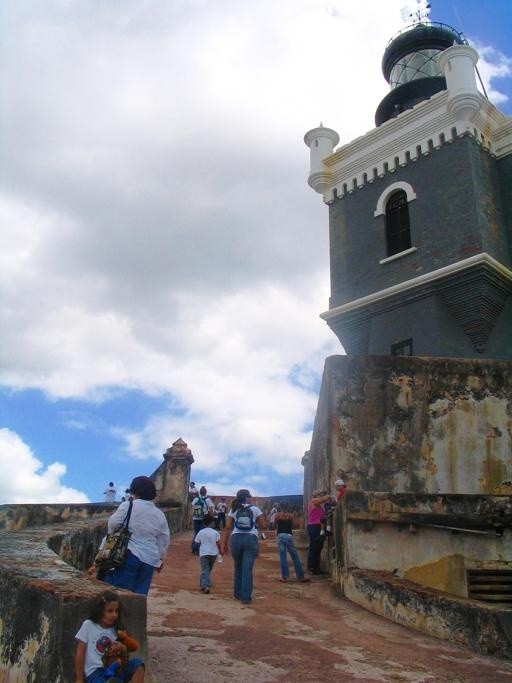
320;526;331;535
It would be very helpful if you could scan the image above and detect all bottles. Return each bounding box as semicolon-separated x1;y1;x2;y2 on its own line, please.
217;554;223;562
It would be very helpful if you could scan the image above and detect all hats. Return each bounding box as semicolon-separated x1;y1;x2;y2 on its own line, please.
335;480;344;486
131;476;154;494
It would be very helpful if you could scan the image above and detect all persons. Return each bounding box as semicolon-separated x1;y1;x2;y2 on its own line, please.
103;482;116;503
221;489;266;604
124;489;131;502
75;589;145;683
335;480;346;501
320;495;338;535
307;490;336;575
194;514;224;594
185;482;302;531
271;500;311;583
191;486;215;555
96;476;171;595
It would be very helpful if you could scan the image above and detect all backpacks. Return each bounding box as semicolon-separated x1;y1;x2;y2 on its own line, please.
192;496;208;516
235;504;254;529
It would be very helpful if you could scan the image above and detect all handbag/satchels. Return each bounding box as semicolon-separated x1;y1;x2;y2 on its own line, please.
95;527;133;567
